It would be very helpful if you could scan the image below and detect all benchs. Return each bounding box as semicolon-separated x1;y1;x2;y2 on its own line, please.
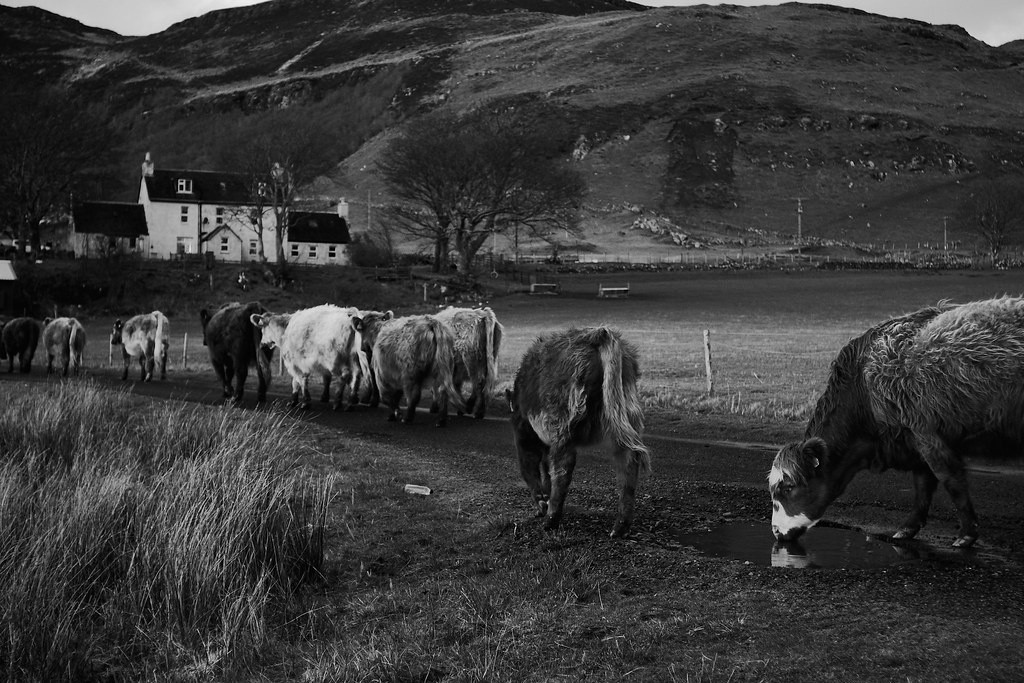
530;283;559;296
597;282;630;300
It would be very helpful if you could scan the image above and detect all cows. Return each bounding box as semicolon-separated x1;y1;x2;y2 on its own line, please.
766;298;1024;552
200;301;506;428
0;317;87;379
504;325;650;539
111;310;171;382
